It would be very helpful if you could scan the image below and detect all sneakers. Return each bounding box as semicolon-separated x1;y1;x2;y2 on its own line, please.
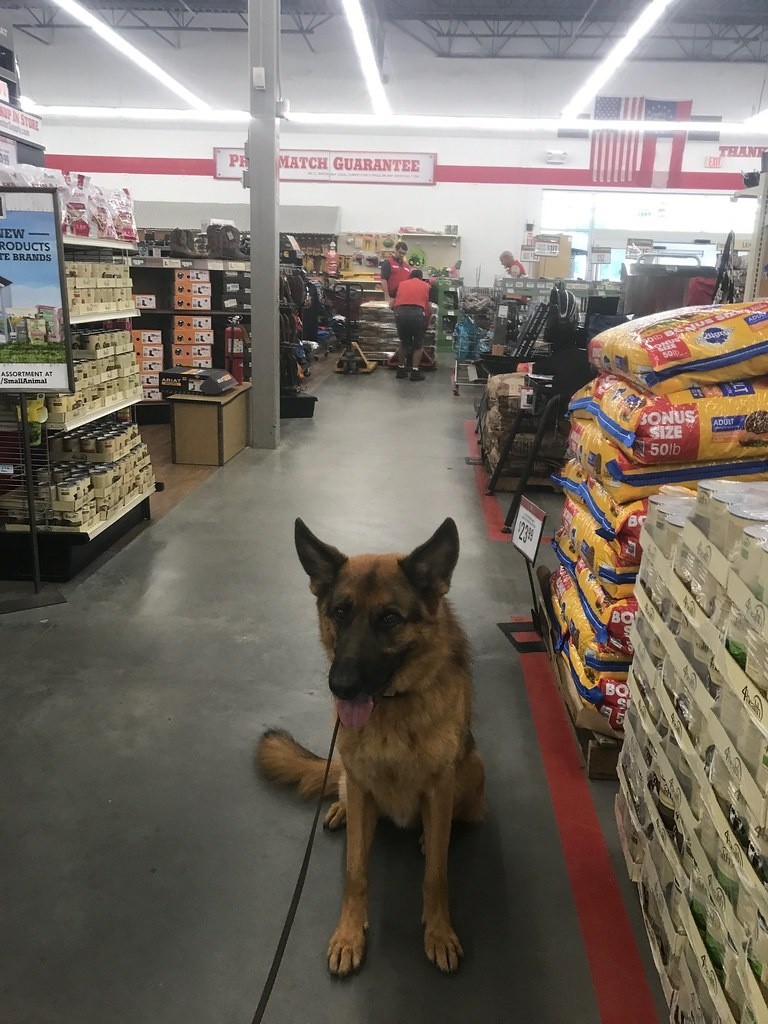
410;370;425;380
396;367;408;377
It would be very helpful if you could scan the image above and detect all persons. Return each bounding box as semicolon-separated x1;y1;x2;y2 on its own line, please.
380;242;413;301
499;250;526;278
394;270;431;381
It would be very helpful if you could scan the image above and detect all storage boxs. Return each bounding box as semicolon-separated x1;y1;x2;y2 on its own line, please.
132;268;239;402
280;391;318;417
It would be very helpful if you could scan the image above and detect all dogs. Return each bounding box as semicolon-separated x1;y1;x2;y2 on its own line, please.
256;515;489;978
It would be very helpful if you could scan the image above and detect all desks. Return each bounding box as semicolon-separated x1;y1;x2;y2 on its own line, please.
166;381;253;466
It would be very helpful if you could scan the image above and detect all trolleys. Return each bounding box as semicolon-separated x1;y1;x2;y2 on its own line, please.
333;281;381;373
383;315;438;371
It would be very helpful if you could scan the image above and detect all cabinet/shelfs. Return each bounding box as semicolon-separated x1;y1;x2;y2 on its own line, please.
130;256;253;425
335;277;386;302
0;233;158;581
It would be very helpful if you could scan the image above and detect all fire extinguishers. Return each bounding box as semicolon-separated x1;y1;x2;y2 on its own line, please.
224;315;246;386
